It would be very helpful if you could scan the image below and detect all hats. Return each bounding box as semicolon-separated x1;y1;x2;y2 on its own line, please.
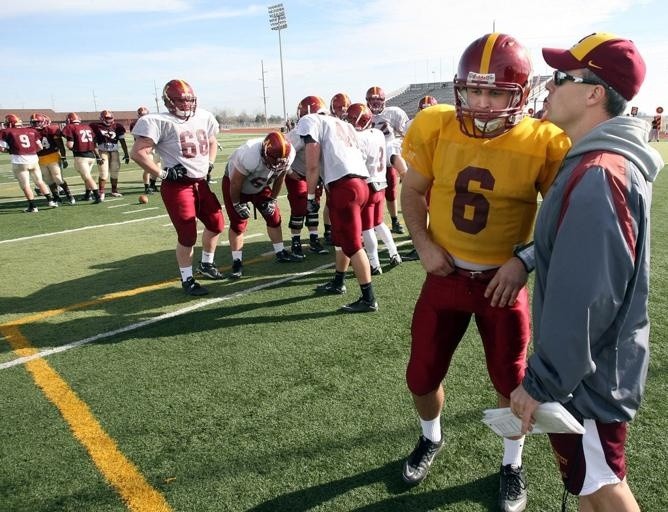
538;31;646;102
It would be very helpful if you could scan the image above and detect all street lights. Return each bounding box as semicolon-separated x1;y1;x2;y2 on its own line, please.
264;2;290;124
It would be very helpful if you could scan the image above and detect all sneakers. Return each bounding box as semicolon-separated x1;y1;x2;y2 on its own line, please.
144;186;160;195
275;229;332;263
400;428;444;484
316;223;421;314
498;463;531;512
181;276;211;296
196;259;244;279
24;185;123;213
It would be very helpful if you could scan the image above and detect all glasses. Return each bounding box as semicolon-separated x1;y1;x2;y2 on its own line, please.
551;69;595;87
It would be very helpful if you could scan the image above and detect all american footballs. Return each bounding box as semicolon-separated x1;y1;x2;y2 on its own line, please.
139;195;148;204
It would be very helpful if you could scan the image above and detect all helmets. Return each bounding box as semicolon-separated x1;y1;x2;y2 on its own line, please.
450;32;531;139
296;86;386;129
261;133;290;173
418;95;436;110
162;79;197;117
3;107;151;127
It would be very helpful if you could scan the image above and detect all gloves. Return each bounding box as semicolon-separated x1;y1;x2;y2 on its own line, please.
122;152;131;164
96;154;105;165
59;157;69;168
306;197;321;212
260;199;276;218
161;162;188;183
232;200;252;219
